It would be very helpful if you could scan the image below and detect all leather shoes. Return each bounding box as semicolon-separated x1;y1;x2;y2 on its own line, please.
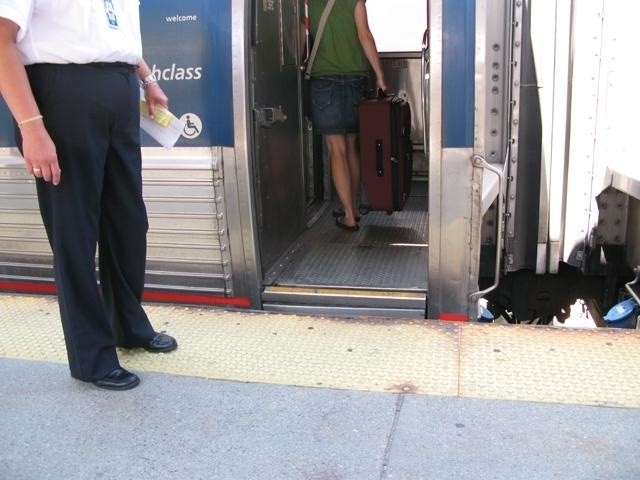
143;333;177;354
93;367;139;391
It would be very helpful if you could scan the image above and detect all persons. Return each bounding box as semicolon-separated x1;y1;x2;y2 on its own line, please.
0;0;177;391
300;0;388;232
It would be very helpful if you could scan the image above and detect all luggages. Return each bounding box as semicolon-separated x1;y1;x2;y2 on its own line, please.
358;86;414;216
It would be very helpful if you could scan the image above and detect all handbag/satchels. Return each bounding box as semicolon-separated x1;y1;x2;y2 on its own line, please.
303;79;310;116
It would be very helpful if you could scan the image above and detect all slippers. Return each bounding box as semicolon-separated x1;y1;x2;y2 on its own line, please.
336;218;359;231
333;207;361;221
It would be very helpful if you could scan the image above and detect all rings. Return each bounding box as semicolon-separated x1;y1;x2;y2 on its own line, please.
33;168;40;171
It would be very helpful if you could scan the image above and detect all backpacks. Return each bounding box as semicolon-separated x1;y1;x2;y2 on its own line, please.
486;262;583;326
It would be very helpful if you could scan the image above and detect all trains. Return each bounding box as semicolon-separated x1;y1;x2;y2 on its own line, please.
0;0;639;329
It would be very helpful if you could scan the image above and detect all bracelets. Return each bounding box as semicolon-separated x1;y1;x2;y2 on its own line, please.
140;73;157;88
17;115;43;125
